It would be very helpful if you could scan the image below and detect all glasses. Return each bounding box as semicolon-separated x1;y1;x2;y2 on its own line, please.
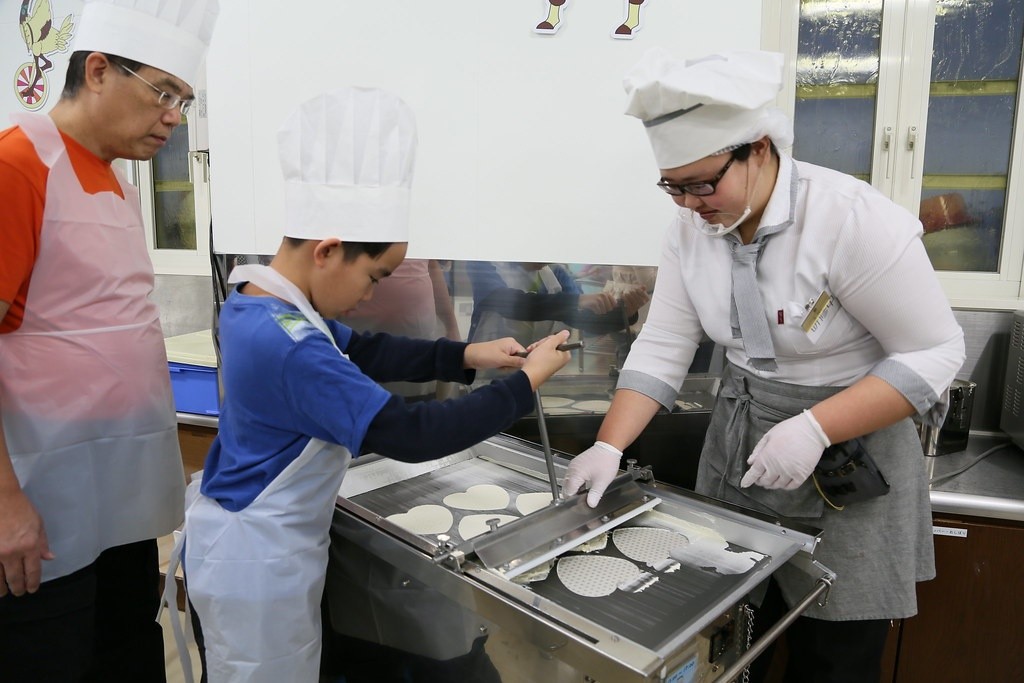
656;142;750;196
108;58;195;118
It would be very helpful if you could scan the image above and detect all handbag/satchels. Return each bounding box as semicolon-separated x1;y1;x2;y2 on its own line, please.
813;433;891;509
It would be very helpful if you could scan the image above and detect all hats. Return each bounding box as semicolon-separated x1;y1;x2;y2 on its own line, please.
277;89;416;242
73;0;221;90
622;50;785;170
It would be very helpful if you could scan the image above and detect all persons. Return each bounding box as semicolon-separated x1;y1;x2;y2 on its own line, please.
159;90;571;683
339;258;659;399
563;48;965;683
0;0;216;683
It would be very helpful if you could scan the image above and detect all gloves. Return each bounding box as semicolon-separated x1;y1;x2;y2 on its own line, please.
559;441;623;508
738;408;831;491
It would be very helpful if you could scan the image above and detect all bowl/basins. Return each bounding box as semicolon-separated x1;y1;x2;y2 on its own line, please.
919;194;974;234
